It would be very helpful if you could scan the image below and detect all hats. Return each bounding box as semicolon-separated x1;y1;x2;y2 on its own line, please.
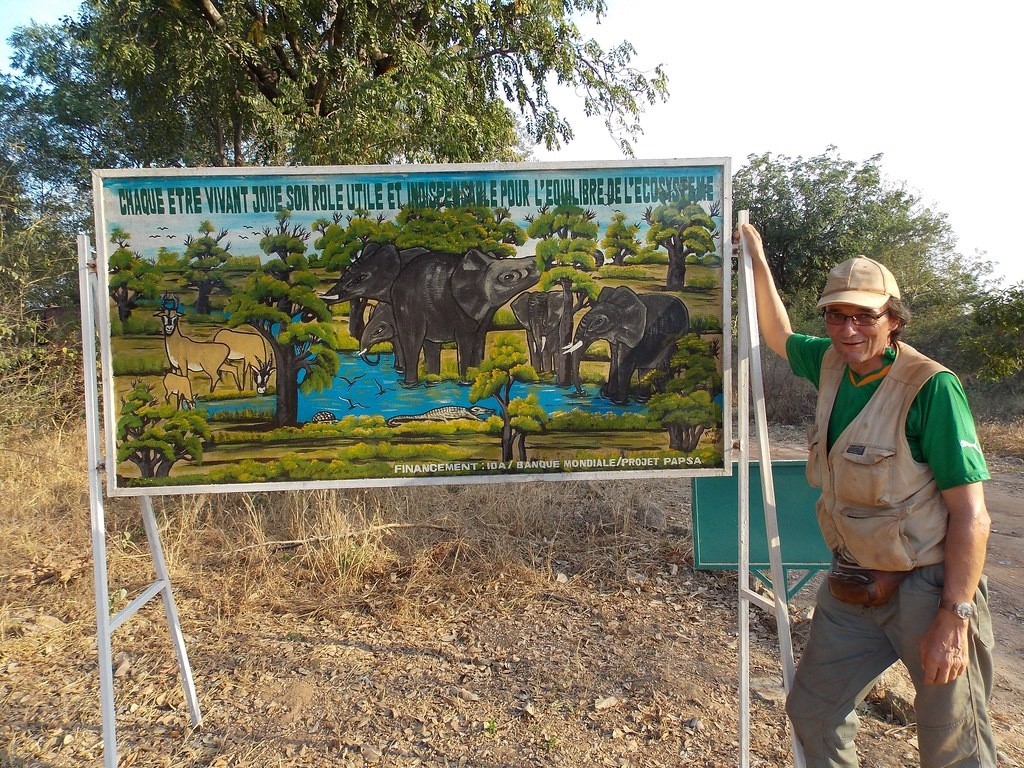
816;255;899;309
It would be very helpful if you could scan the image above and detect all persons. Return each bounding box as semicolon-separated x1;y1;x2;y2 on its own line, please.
731;220;999;768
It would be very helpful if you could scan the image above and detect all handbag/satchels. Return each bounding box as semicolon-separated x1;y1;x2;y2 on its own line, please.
828;557;913;606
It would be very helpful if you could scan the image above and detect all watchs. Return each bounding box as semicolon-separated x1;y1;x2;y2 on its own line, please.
937;598;974;620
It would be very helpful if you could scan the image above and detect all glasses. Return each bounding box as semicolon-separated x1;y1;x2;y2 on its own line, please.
822;308;889;325
